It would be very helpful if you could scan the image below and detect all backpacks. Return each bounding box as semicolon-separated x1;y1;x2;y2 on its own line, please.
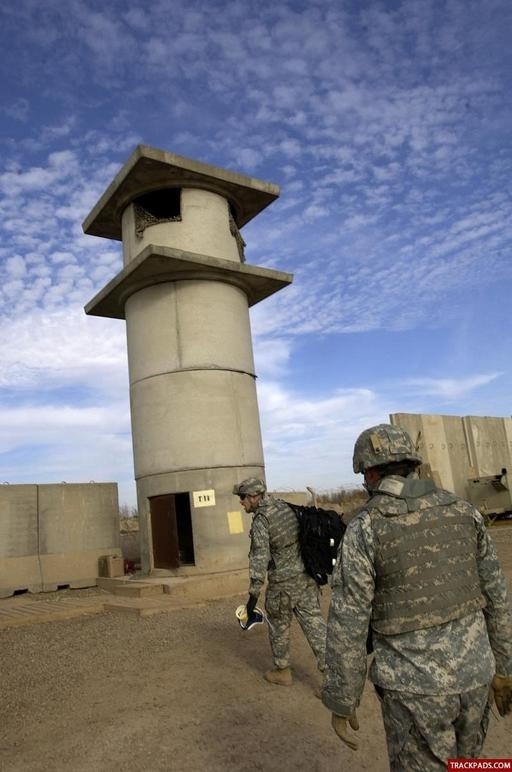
285;502;347;585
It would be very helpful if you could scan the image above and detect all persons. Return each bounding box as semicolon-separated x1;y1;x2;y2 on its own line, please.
317;424;512;772
230;476;330;699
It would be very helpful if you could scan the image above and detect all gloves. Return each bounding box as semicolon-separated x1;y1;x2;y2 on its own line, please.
247;595;258;616
492;674;512;717
331;712;360;751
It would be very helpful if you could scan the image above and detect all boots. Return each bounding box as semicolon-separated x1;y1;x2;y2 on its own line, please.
264;665;293;686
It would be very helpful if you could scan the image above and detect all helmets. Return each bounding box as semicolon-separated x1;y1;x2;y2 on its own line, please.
353;424;423;473
233;477;266;496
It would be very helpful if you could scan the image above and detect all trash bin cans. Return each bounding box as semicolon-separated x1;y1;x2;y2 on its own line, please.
99;554;125;578
